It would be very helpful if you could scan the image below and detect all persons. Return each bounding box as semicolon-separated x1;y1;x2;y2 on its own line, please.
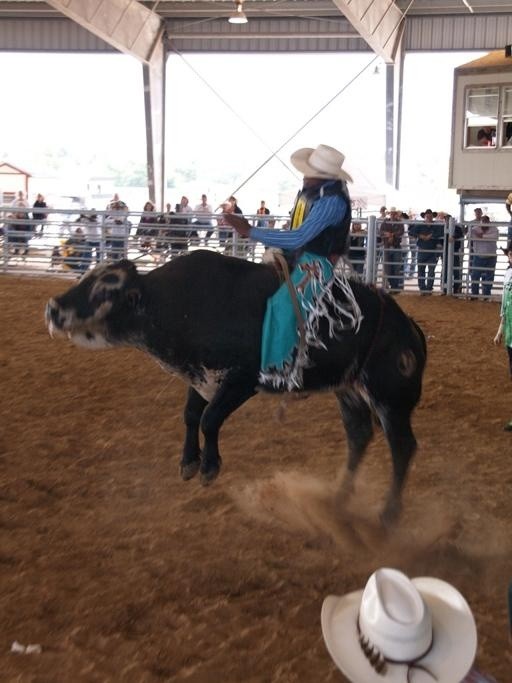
222;142;366;393
493;242;512;432
0;191;511;302
477;122;510;148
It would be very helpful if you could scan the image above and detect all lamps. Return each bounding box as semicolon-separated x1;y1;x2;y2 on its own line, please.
228;0;248;25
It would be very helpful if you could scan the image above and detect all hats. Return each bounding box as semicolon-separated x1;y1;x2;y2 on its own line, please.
420;209;437;218
291;146;353;183
385;207;401;217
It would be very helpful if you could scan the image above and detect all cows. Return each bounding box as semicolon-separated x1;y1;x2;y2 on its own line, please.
45;250;427;532
